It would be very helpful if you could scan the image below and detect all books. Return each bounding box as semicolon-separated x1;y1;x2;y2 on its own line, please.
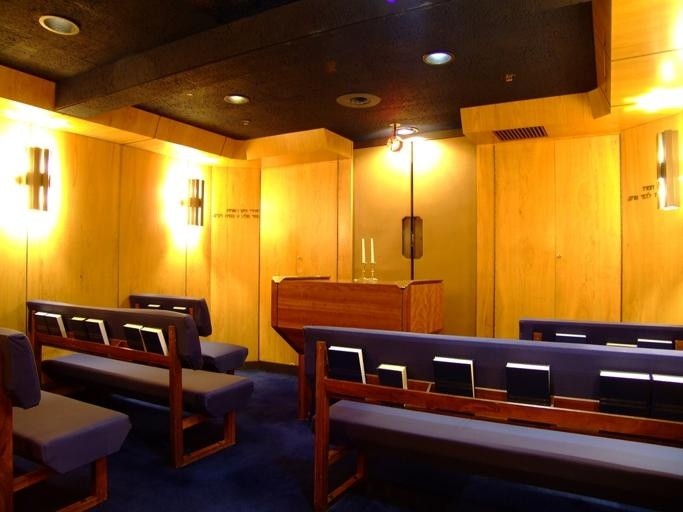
146;303;159;308
555;333;587;344
505;361;552;426
123;323;145;351
44;313;66;338
650;373;682;422
377;363;408;407
433;356;476;416
33;311;49;334
327;345;366;400
84;318;109;345
172;306;186;312
598;370;651;417
637;338;672;349
606;342;636;348
140;327;167;356
70;316;88;341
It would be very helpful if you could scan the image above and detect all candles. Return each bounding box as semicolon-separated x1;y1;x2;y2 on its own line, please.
371;237;374;263
361;238;365;264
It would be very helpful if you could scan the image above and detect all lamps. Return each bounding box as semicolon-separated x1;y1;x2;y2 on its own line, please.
16;147;51;212
386;121;404;153
655;129;683;211
180;178;205;227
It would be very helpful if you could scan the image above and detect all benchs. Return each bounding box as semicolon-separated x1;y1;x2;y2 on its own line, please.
0;327;131;512
301;325;683;512
129;294;248;375
519;319;683;350
26;298;254;469
